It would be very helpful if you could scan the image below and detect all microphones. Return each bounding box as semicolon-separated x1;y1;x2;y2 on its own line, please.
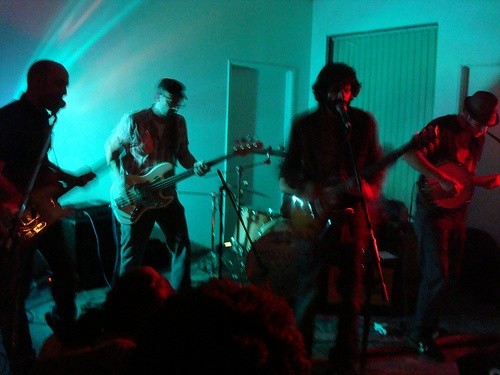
336;100;351;129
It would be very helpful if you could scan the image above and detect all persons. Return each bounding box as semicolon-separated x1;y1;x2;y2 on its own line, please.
281;61;388;375
103;78;210;291
0;59;97;375
403;90;500;364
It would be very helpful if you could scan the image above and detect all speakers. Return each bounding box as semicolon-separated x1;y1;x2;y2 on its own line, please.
60;215;115;288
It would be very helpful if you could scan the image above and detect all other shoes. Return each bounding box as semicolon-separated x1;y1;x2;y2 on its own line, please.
407;333;444;362
45;306;75;334
434;328;448;335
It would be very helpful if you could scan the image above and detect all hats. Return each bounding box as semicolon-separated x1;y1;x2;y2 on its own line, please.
463;91;500;127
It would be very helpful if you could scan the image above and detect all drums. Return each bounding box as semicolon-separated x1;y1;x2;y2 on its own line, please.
244;218;307;294
234;205;284;250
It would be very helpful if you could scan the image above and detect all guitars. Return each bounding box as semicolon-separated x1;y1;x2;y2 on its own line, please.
110;135;262;226
2;139;131;243
289;126;442;240
417;158;500;216
